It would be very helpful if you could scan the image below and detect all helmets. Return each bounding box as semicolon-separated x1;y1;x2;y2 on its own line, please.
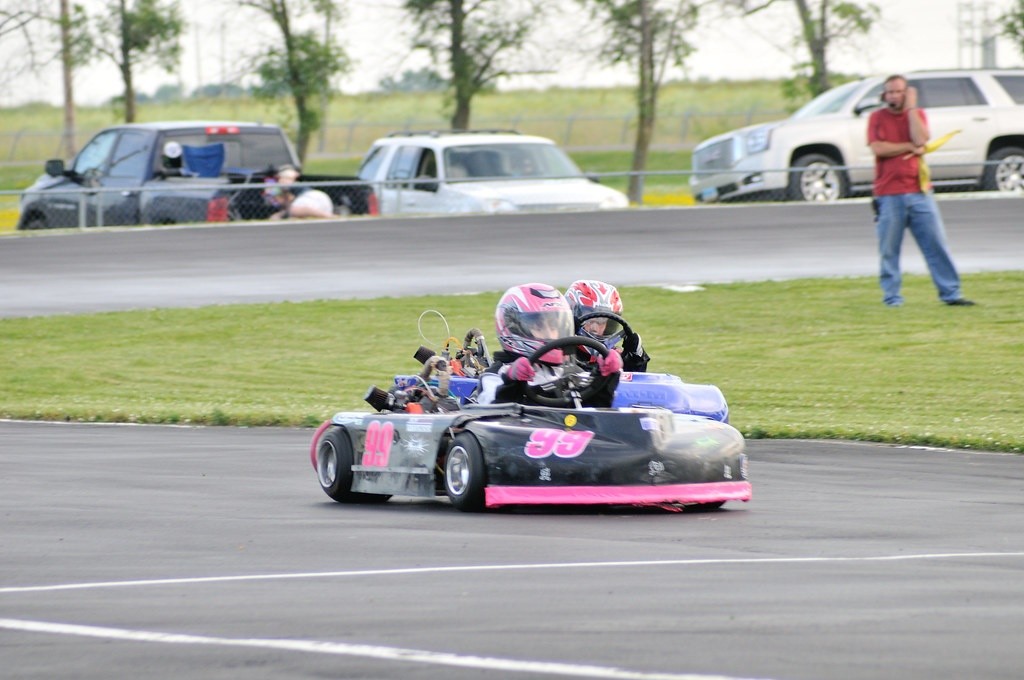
564;280;624;354
495;282;574;364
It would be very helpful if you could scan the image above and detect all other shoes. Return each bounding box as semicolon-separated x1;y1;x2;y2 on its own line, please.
947;299;975;306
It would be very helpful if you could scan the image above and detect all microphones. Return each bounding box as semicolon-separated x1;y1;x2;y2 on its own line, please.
888;104;896;107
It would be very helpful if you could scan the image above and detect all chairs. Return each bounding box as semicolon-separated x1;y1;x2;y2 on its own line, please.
183;145;256;221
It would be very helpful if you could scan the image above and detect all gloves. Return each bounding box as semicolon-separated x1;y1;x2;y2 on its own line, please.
597;348;623;376
506;356;535;382
622;332;642;355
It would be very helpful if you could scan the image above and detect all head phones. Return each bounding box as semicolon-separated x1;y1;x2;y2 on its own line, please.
881;92;886;102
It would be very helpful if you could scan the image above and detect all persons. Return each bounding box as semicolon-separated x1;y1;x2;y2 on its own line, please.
868;76;979;307
477;279;650;408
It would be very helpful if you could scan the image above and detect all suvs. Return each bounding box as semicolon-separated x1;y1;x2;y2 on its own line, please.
355;128;630;212
693;66;1024;201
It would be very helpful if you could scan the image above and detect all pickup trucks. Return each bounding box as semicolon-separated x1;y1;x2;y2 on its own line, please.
16;123;382;230
